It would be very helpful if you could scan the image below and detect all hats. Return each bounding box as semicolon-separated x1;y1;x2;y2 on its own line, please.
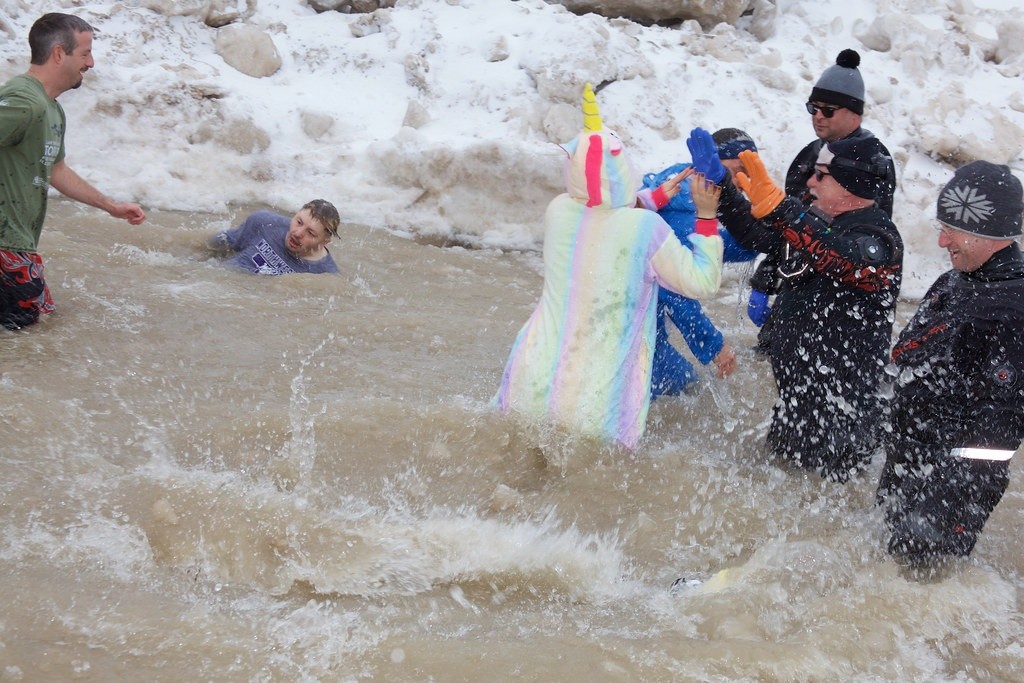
937;160;1023;239
815;138;882;177
810;49;864;115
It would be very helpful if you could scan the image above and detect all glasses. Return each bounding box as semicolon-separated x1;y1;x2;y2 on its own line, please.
805;100;846;118
814;168;832;182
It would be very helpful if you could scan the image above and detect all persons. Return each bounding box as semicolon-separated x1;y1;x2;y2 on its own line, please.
747;47;898;358
687;126;905;481
876;162;1024;557
0;13;146;332
491;84;725;451
630;127;761;399
209;198;343;274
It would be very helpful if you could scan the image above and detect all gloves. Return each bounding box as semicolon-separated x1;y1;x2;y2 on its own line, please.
735;149;786;220
686;127;726;187
